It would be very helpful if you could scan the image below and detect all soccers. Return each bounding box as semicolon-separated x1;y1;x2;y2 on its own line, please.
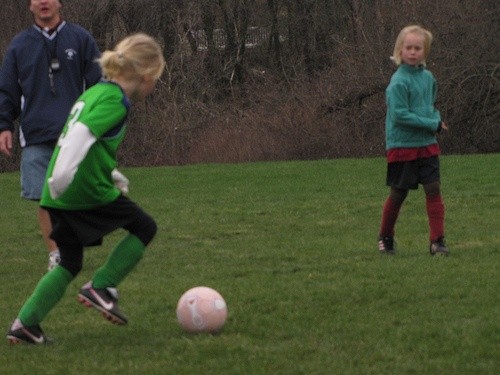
176;286;228;334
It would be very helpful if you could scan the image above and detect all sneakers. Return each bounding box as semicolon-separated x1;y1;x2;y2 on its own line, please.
48;249;61;271
77;280;128;325
7;317;55;344
430;236;448;255
377;232;394;254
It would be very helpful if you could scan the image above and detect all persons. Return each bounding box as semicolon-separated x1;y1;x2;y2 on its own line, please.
0;0;105;272
5;33;166;347
376;25;450;257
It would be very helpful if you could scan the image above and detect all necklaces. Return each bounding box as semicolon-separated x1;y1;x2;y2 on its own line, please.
39;28;61;88
